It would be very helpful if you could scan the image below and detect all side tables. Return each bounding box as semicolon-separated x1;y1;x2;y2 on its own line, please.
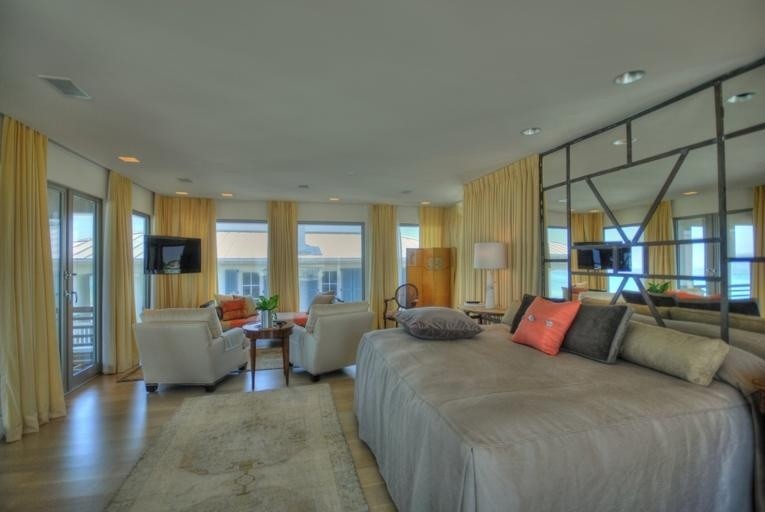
242;320;294;391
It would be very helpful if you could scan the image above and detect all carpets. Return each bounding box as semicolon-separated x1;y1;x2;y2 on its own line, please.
101;382;370;512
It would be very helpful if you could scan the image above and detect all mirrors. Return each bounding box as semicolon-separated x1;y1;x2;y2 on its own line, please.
540;56;765;316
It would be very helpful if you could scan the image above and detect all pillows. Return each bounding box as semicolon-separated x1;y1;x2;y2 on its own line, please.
222;298;245;321
233;295;258;316
502;289;765;395
622;290;676;306
393;306;485;340
679;297;761;316
214;294;233;304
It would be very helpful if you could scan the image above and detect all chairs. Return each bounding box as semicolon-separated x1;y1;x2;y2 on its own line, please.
289;301;376;382
383;283;419;329
132;308;251;392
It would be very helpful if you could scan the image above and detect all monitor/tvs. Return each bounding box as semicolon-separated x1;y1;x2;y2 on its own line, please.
144;234;201;274
575;241;632;271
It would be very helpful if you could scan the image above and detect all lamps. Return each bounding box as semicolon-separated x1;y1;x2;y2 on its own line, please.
473;241;508;309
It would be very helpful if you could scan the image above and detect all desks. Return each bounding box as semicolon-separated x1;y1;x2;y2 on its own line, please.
462;306;506;325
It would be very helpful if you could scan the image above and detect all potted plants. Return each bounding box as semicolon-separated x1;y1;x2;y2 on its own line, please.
255;294;281;328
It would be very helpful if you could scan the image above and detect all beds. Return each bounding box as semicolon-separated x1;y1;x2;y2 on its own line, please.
199;293;336;332
352;324;764;512
